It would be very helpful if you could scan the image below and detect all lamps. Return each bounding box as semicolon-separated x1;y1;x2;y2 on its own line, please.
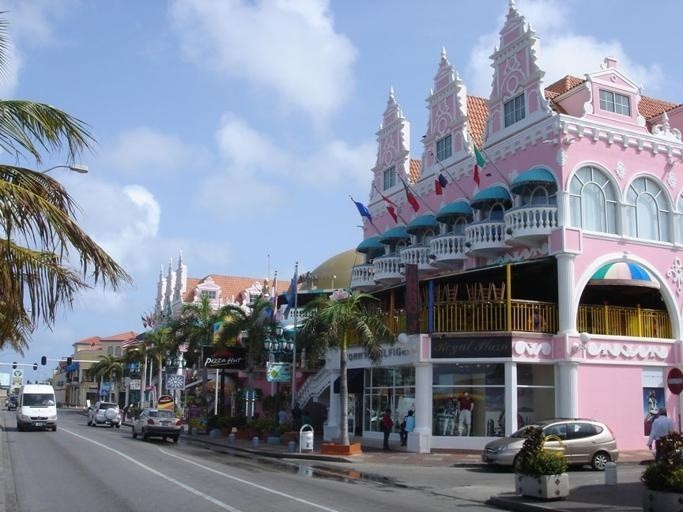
398;327;414;344
572;332;589;352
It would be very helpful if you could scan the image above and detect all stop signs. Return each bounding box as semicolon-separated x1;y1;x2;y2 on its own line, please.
667;368;683;394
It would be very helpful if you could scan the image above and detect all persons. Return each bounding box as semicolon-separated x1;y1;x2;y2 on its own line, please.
291;402;302;421
645;407;674;460
398;409;415;446
299;410;312;427
441;397;456;436
277;407;290;427
456;390;475;435
381;406;393;450
41;394;53;406
528;309;543;332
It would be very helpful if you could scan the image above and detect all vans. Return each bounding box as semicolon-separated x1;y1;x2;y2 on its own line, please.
17;384;57;431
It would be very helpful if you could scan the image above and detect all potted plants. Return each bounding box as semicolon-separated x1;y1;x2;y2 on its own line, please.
641;431;683;512
240;419;273;440
515;426;570;501
218;415;247;436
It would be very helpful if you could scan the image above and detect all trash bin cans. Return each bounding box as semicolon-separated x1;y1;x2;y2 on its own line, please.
299;424;314;453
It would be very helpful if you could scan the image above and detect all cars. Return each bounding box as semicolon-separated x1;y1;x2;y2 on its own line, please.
87;401;120;428
5;396;18;411
482;418;619;471
132;408;184;442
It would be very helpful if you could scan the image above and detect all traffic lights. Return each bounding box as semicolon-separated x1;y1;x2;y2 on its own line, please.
67;357;72;365
41;356;46;365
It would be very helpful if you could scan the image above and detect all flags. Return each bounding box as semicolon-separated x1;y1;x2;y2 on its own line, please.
375;187;397;224
349;196;372;225
469;139;487;197
282;264;297;320
140;316;146;328
397;175;419;213
144;313;152;327
260;275;276;325
430;154;453;198
